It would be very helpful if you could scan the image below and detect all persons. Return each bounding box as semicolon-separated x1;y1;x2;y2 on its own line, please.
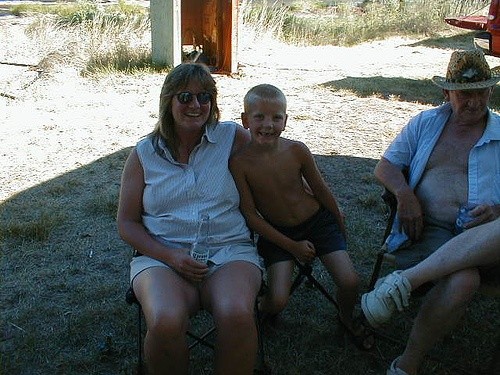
361;50;500;375
230;85;376;375
116;63;313;375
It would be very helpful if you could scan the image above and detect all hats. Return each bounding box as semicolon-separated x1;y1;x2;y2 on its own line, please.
431;49;500;90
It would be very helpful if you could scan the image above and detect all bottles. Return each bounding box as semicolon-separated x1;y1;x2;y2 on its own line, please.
189;213;210;264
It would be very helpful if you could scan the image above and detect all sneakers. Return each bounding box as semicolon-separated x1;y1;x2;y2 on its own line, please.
387;355;407;375
361;270;412;329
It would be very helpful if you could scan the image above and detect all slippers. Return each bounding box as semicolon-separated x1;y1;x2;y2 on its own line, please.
337;313;376;352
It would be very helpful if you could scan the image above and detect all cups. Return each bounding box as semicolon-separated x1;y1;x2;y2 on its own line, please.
455;202;480;233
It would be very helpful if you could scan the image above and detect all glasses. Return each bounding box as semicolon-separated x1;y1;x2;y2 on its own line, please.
173;91;211;104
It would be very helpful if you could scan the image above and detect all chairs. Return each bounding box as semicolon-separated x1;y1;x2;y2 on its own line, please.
362;164;500;375
445;0;500;56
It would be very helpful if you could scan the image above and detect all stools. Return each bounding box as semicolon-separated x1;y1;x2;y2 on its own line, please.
125;274;267;375
261;257;342;328
